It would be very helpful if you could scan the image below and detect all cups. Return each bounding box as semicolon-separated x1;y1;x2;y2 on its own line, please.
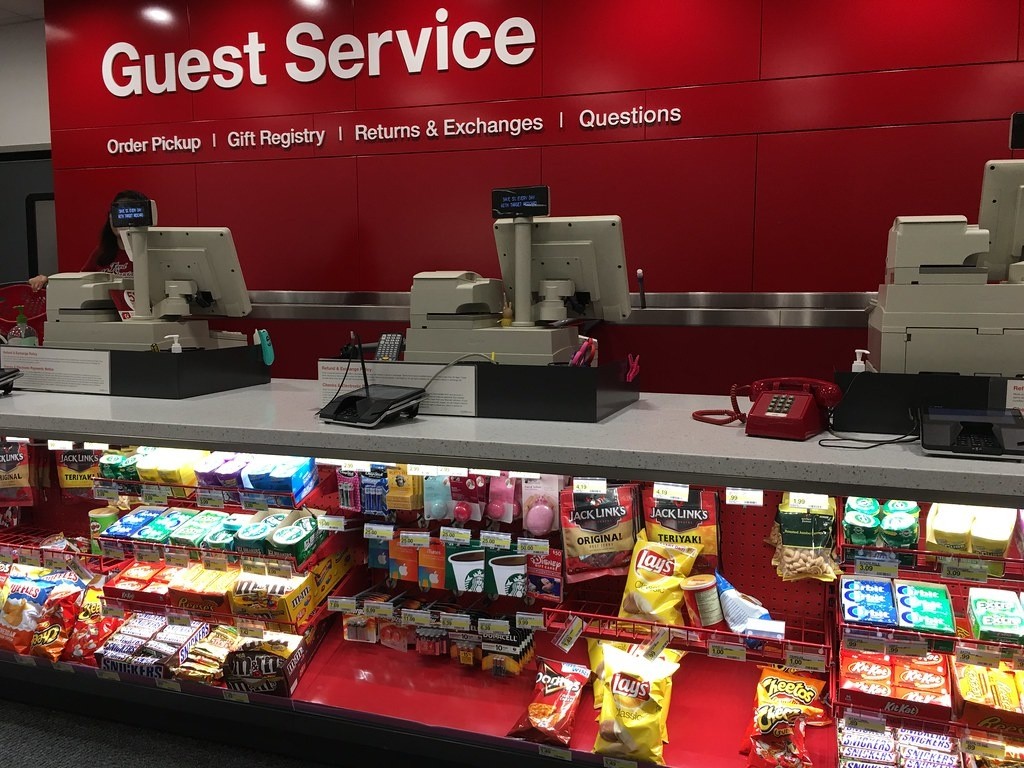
448;550;484;591
488;554;527;598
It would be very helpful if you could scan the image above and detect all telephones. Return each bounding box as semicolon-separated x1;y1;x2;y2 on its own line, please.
742;374;840;440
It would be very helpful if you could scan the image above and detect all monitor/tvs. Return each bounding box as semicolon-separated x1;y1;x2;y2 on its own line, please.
126;227;252;317
493;215;631;321
318;382;425;428
978;159;1024;282
918;406;1024;460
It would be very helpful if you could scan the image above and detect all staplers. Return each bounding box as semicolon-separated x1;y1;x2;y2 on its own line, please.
253;329;275;366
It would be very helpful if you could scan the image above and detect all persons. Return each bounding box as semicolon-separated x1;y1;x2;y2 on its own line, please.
28;190;157;320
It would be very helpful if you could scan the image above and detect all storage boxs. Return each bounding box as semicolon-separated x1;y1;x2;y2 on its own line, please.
100;505;170;558
204;513;254;564
170;509;230;560
102;559;187;615
228;548;356;633
167;564;233;625
233;508;292;556
94;622;213;679
948;655;1024;741
838;641;951;732
132;507;200;560
213;613;337;697
264;507;329;568
839;574;956;653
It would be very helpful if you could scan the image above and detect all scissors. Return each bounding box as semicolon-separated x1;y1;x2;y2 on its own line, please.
570;337;596;367
